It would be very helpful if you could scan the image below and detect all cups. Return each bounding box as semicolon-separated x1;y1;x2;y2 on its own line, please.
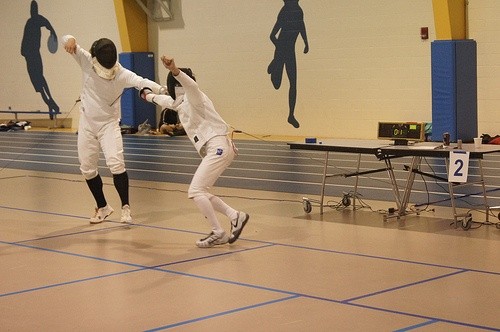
474;137;483;148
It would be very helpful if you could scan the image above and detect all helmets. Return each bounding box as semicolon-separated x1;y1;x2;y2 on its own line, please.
167;66;196;100
90;38;120;79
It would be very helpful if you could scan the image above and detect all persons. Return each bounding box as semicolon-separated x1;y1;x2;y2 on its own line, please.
62;34;170;224
141;55;249;248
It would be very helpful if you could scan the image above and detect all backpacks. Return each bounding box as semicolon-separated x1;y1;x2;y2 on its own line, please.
158;108;178;132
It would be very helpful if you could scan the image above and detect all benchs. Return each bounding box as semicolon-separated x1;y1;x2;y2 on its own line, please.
0;111;62;128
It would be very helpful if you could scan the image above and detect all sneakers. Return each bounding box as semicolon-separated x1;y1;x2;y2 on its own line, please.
121;204;133;223
89;201;115;223
228;210;249;243
196;230;229;248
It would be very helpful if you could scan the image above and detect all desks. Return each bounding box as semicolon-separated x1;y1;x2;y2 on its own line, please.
381;143;500;229
288;139;421;222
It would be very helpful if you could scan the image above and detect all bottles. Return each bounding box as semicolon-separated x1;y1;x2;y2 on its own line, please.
457;136;462;149
443;131;449;146
